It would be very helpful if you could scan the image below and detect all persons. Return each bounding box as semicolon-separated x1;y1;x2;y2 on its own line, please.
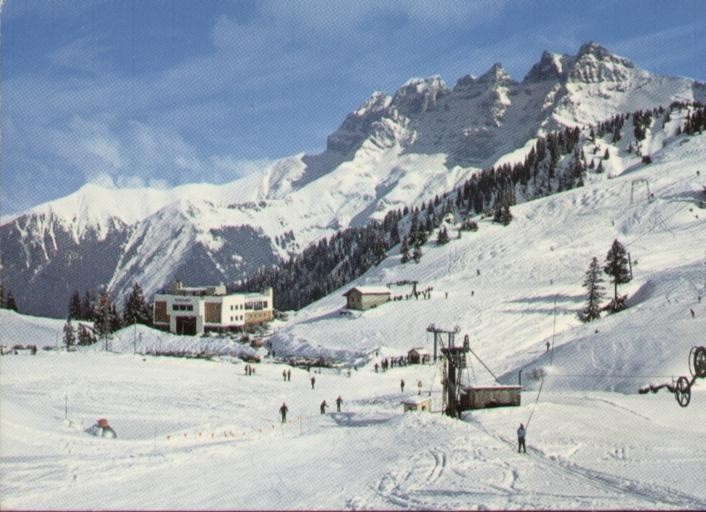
233;337;434;396
278;401;288;421
319;399;328;416
516;422;528;454
336;395;343;411
